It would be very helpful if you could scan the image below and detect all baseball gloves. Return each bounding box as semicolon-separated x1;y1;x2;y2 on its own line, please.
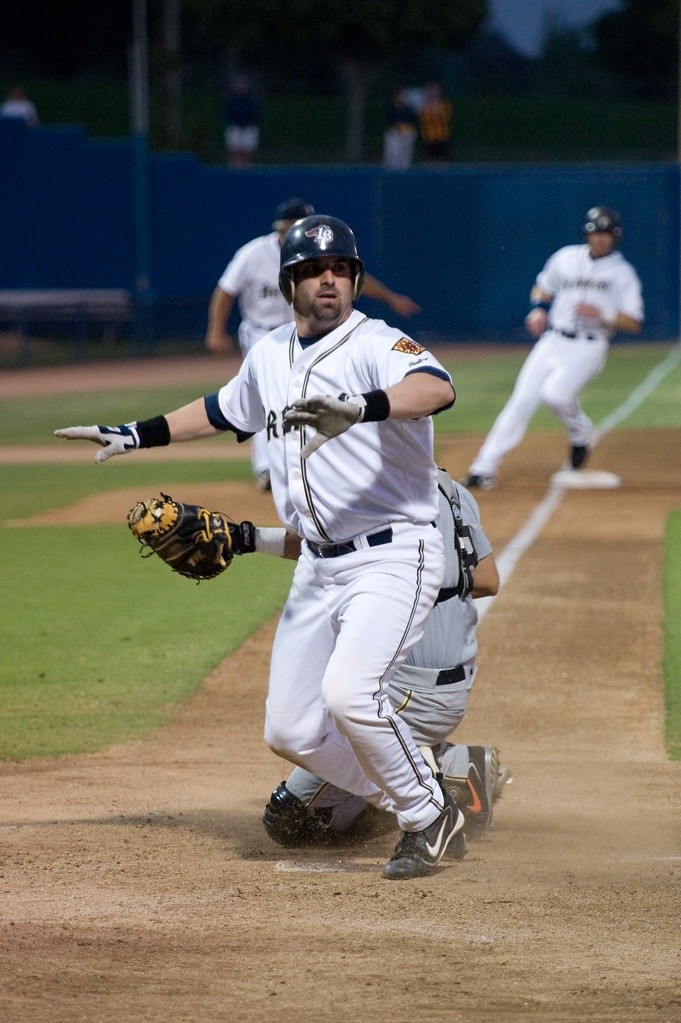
125;492;261;586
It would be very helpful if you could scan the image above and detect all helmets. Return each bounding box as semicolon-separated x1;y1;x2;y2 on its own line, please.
278;213;365;307
583;205;621;233
275;198;315;219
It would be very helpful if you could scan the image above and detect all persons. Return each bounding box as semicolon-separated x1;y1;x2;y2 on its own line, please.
56;215;468;879
0;86;41;126
128;465;505;849
207;199;419;490
457;207;645;490
375;83;455;173
219;74;265;160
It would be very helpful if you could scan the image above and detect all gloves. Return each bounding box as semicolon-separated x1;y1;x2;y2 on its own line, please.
54;420;141;465
282;394;368;462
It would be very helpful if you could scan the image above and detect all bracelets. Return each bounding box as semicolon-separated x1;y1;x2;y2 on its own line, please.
529;299;550;311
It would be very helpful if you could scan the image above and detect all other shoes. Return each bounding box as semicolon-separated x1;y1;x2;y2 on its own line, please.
461;474;498;491
571;445;588;469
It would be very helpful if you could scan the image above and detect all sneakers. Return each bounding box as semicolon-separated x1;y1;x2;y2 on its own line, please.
382;770;465;880
444;745;510;845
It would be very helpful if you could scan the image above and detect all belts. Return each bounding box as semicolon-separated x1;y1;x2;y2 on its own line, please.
548;326;596;340
436;665;473;685
304;520;437;559
